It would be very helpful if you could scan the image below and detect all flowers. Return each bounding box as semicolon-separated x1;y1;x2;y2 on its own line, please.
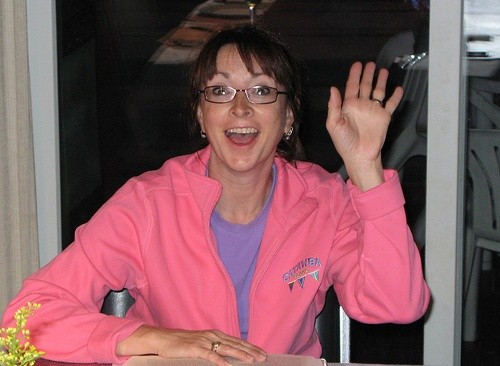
0;302;45;366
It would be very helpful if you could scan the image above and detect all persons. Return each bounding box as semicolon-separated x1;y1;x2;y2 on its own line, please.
0;25;432;366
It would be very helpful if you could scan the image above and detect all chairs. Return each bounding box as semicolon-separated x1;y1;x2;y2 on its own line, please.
101;284;339;366
337;52;500;341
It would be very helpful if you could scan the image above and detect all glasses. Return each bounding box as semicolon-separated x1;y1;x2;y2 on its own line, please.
197;84;295;104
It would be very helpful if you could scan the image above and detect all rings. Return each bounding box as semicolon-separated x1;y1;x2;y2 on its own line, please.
211;341;222;353
372;98;384;107
